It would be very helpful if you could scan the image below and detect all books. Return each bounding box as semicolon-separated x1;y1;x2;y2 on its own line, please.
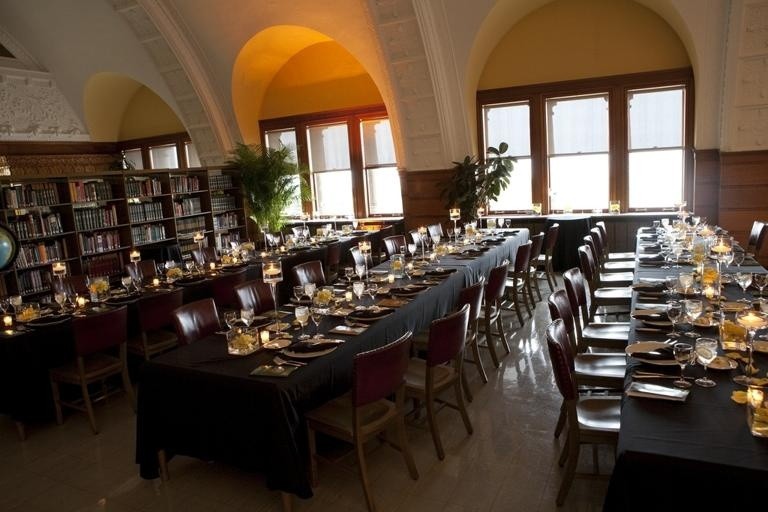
19;271;52;295
84;254;120;279
171;176;199;192
69;181;114;203
222;232;240;249
74;206;117;231
5;183;59;209
179;237;208;255
210;196;234;211
208;175;233;189
129;202;163;223
214;213;238;230
177;217;206;235
9;210;64;239
174;198;201;216
79;231;120;255
127;180;161;198
133;224;165;244
50;263;71;280
15;241;61;268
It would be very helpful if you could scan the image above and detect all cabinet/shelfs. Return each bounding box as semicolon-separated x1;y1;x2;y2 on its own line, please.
0;164;251;314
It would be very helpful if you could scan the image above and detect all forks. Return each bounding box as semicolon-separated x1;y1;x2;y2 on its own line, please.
273;356;307;367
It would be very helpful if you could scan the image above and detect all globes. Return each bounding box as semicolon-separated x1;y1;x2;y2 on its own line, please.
0;224;18;272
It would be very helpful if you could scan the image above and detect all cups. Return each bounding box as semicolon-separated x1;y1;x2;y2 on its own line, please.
755;274;768;301
719;310;748;351
358;241;372;295
355;265;366;282
745;385;768;437
679;273;693;303
154;261;174;287
311;309;325;339
294;286;304;306
295;306;310;340
368;284;378;308
653;215;734;269
226;326;259;356
284;214;353;241
531;203;542;216
695;338;718;387
737;272;752;303
224;311;236;330
24;302;41;319
667;304;681;338
685;300;702;337
666;276;678;304
68;293;78;313
734;252;743;275
55;291;68;312
721;255;732;277
609;200;620;214
400;207;511;266
0;294;25;331
262;262;293;339
121;251;142;296
345;267;353;289
185;227;214;276
91;275;110;302
305;283;316;306
241;308;255;328
701;259;721;311
217;218;284;257
673;344;693;387
353;282;366;311
390;253;405;279
52;261;66;291
733;310;768;388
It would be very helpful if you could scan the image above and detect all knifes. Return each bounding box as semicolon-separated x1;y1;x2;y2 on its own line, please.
635;370;670;376
631;374;692;379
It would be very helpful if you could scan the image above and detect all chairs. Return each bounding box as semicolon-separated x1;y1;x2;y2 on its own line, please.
381;235;408;259
582;235;634;285
578;244;632;319
190;246;217;265
128;287;184;359
507;240;533;327
563;268;630;352
478;262;511;368
547;289;627;439
291;225;312;242
408;228;429;249
380;225;396;238
412;282;489;402
596;221;636;261
351;234;372;248
589;227;635;271
170;298;223;345
404;303;474;461
745;218;768;255
212;269;248;310
426;222;444;244
123;260;158;282
547;317;622;505
531;223;559;291
48;274;92;301
232;277;277;317
345;246;373;272
302;328;419;512
291;261;327;295
508;231;543;309
323;242;342;283
46;305;137;434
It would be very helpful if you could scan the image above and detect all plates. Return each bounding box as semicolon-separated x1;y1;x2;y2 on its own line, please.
221;229;312;265
425;268;458;277
264;340;291;349
448;229;520;260
285;338;339;357
631;310;673;325
633;285;668;296
391;285;429;296
224;316;275;330
329;308;353;316
290;294;317;303
707;226;755;258
674;287;701;295
347;306;394;320
320;227;367;243
688;317;719;327
718;301;753;313
23;314;75;328
174;260;247;287
390;226;472;257
330;277;348;287
105;295;145;305
698;356;738;370
324;288;346;295
625;342;678;365
639;229;668;267
